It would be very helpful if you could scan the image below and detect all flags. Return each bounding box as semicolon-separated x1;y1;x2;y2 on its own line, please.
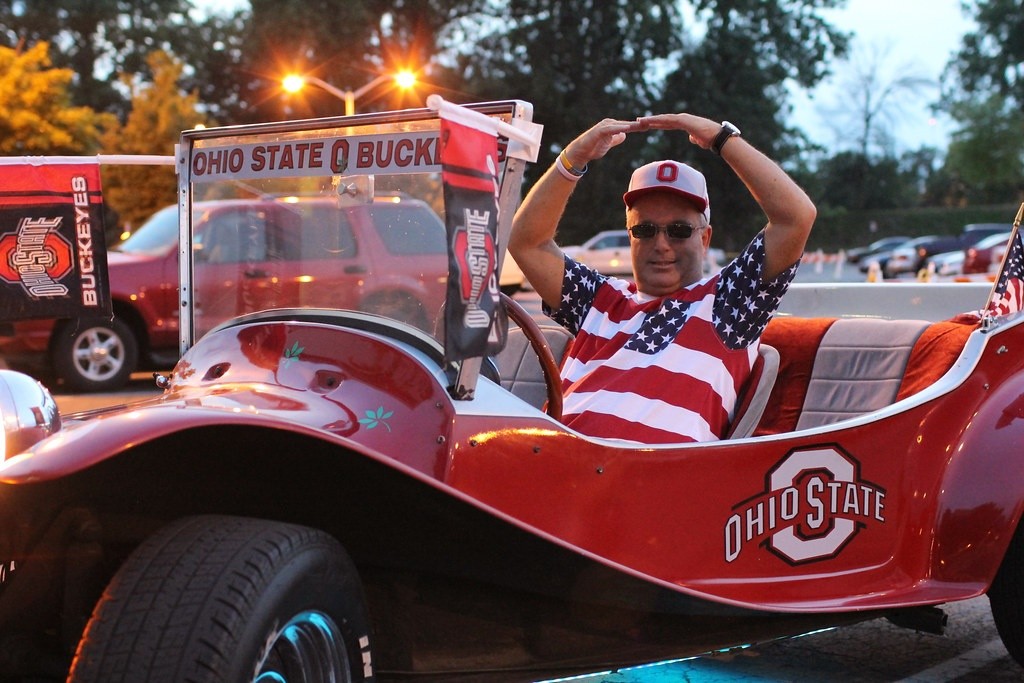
988;230;1024;318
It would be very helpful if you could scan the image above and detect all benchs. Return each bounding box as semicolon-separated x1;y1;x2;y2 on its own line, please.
752;317;977;442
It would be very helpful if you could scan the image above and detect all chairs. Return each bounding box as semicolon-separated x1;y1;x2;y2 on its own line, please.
726;339;779;441
487;321;574;410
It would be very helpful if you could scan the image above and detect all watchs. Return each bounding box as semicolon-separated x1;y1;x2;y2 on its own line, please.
710;120;742;156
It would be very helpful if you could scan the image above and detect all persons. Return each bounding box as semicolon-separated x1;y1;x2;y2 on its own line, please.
509;114;817;443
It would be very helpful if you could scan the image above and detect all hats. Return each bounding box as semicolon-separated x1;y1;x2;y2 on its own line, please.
623;160;710;224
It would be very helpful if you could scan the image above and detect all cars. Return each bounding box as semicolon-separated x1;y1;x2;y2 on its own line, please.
846;237;913;264
962;239;1010;274
887;248;916;271
927;232;1024;275
913;223;1024;272
558;231;634;275
857;236;938;273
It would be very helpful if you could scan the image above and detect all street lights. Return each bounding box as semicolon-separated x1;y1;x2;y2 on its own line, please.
282;67;418;117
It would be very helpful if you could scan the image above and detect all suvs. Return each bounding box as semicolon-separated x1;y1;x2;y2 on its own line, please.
1;191;449;390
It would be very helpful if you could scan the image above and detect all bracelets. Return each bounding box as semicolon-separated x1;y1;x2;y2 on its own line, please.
561;149;588;175
556;155;583;181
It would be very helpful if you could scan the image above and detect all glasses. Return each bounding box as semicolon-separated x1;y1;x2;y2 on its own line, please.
627;224;705;239
